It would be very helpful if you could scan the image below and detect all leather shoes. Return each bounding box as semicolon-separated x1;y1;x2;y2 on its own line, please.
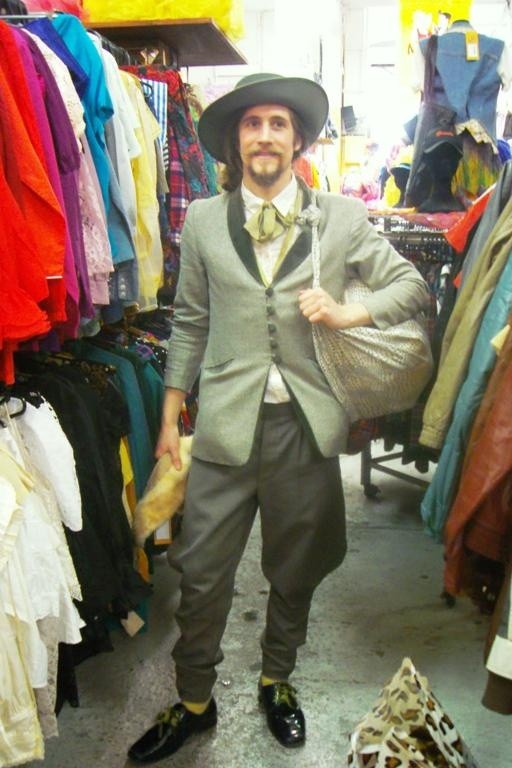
257;676;305;748
128;696;217;763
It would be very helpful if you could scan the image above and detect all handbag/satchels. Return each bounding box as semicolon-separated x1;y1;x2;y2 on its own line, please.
312;278;436;422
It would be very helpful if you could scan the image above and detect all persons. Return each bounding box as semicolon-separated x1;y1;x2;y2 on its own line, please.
124;70;433;764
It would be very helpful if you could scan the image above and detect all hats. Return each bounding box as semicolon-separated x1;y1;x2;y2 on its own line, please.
198;72;329;165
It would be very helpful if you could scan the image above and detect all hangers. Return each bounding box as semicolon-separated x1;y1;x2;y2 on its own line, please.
0;311;180;428
93;29;142;66
143;83;153;98
392;221;456;269
